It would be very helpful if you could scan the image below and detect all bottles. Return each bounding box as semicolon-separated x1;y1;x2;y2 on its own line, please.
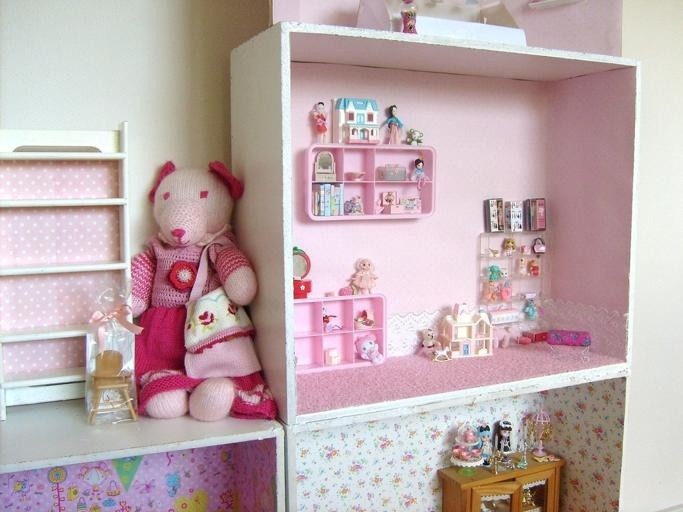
399;0;419;35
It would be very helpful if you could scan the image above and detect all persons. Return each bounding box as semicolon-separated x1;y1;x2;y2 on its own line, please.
450;402;556;477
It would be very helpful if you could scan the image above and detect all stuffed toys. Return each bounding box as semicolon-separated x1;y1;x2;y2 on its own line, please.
127;160;278;421
